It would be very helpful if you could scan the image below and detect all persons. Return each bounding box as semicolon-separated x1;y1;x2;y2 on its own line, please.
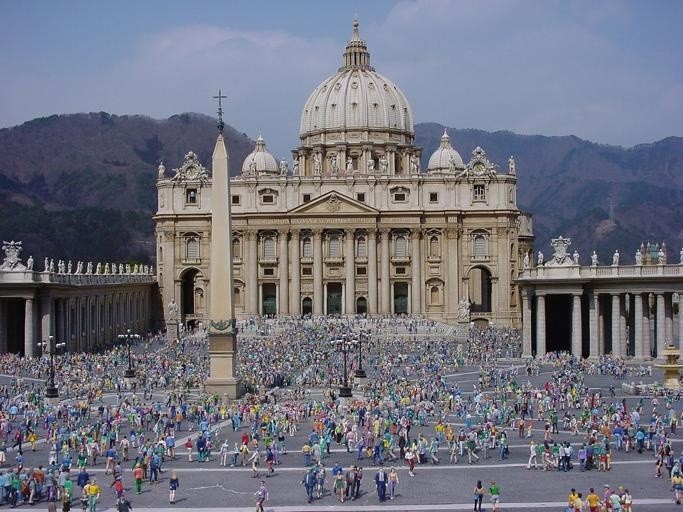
680;248;683;264
45;257;153;275
168;299;179;316
248;154;455;176
508;155;515;173
456;296;467;319
158;162;165;179
538;252;544;265
0;313;682;512
27;256;34;270
573;249;664;265
